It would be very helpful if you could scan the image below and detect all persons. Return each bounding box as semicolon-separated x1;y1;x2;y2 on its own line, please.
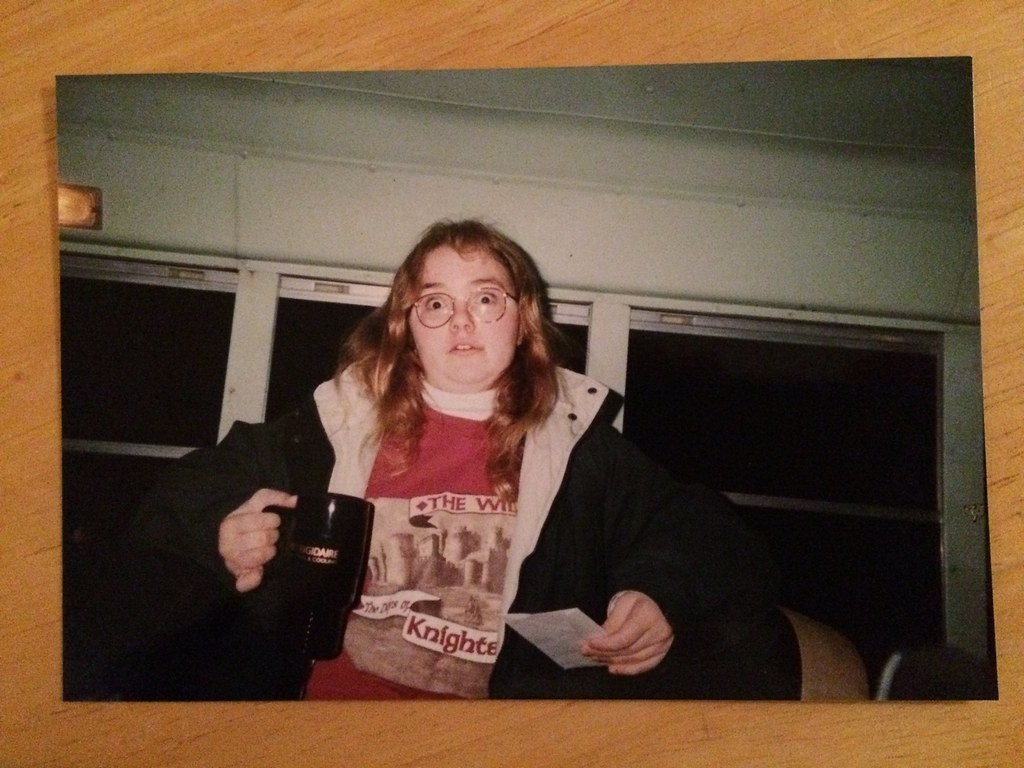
123;219;762;702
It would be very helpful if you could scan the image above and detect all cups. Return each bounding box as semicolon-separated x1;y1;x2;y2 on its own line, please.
260;491;373;658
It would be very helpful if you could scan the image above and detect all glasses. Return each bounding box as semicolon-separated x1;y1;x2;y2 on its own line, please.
405;286;520;328
